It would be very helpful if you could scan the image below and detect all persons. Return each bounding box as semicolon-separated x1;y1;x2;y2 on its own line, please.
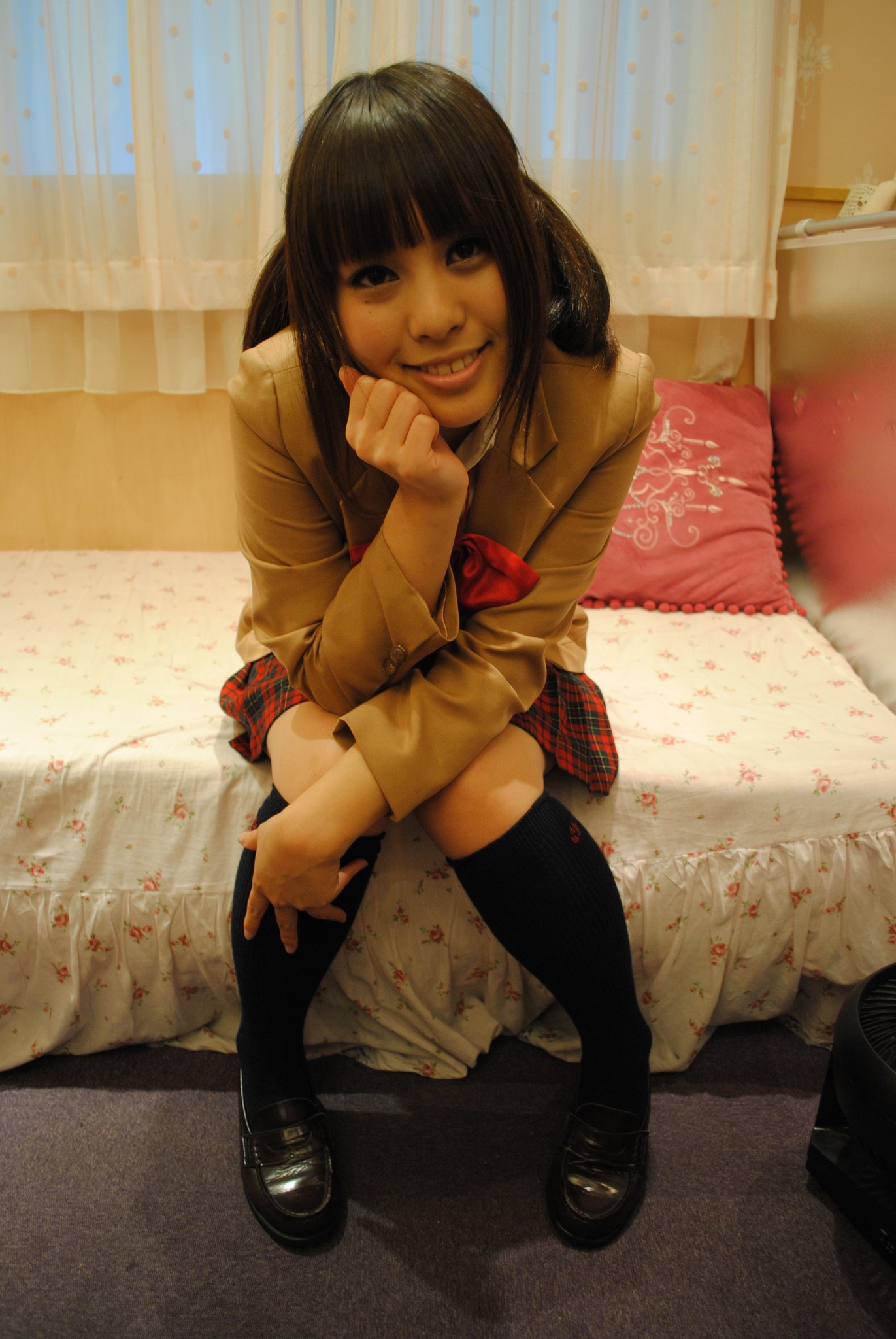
219;61;657;1242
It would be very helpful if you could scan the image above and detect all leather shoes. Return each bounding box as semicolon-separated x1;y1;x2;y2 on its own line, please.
544;1093;651;1253
236;1065;334;1249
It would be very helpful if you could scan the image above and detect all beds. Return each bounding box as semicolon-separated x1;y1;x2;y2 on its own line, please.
0;209;896;1078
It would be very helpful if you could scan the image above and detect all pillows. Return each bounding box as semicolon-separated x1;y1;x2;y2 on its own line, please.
582;378;808;616
